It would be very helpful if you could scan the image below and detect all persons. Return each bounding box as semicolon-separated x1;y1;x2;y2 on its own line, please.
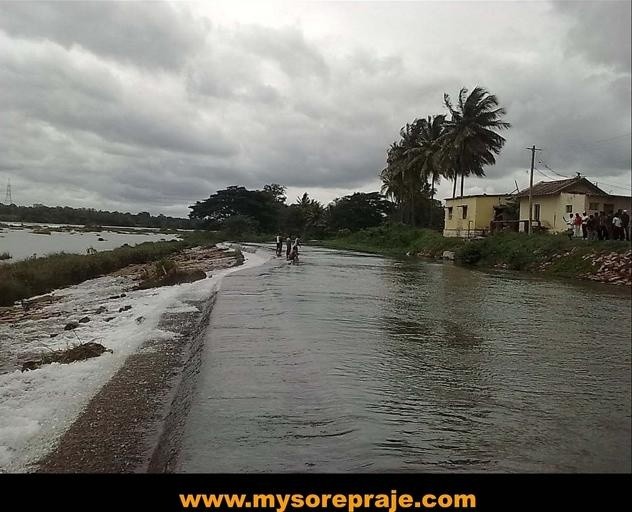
274;232;301;260
562;209;631;240
